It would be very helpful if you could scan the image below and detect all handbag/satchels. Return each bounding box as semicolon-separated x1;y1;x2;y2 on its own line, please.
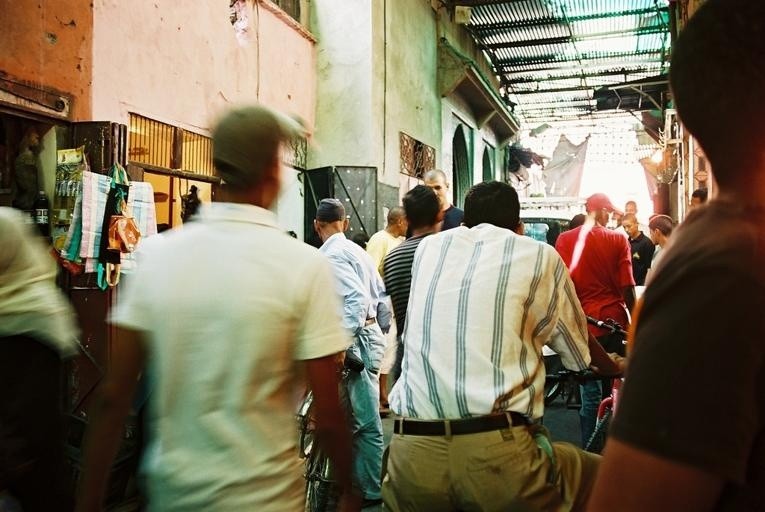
60;164;158;274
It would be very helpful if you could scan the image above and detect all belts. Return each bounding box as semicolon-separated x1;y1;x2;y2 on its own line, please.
365;318;378;325
393;412;524;435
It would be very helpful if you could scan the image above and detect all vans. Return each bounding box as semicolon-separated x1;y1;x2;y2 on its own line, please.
499;192;588;392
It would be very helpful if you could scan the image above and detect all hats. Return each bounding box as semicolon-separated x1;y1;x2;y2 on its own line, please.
212;106;310;176
315;199;345;221
587;194;616;212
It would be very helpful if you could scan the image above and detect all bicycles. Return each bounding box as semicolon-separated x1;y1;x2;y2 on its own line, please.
296;355;366;512
581;308;631;456
537;364;601;426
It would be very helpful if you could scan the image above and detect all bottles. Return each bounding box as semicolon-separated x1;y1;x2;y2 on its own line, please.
35;190;49;236
